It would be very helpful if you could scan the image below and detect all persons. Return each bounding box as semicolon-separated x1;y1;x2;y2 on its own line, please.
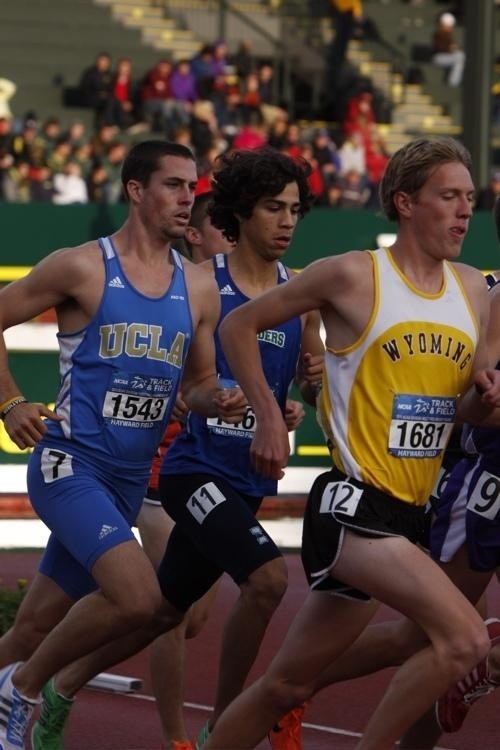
0;137;499;750
318;0;469;87
0;39;394;206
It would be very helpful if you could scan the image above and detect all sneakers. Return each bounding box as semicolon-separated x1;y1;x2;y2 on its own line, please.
0;662;78;749
266;700;308;750
434;616;500;734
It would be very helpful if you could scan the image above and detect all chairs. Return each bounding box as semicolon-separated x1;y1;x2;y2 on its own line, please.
1;2;499;133
